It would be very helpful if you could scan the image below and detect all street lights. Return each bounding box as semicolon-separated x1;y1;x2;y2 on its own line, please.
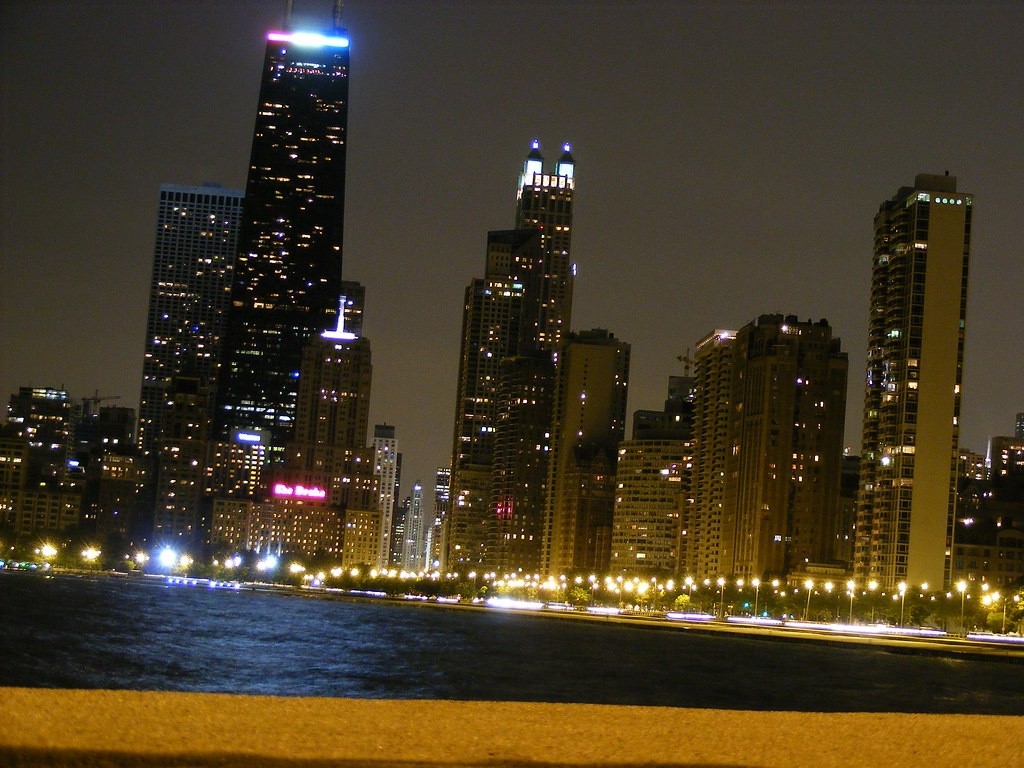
867;581;878;624
897;581;908;626
803;580;813;621
666;580;675;611
847;579;856;625
616;575;622;609
684;576;692;612
718;577;725;619
752;578;761;619
588;575;595;605
956;580;968;630
651;577;657;611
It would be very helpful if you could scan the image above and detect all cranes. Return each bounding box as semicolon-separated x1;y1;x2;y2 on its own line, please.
675;347;696;376
81;390;120;414
